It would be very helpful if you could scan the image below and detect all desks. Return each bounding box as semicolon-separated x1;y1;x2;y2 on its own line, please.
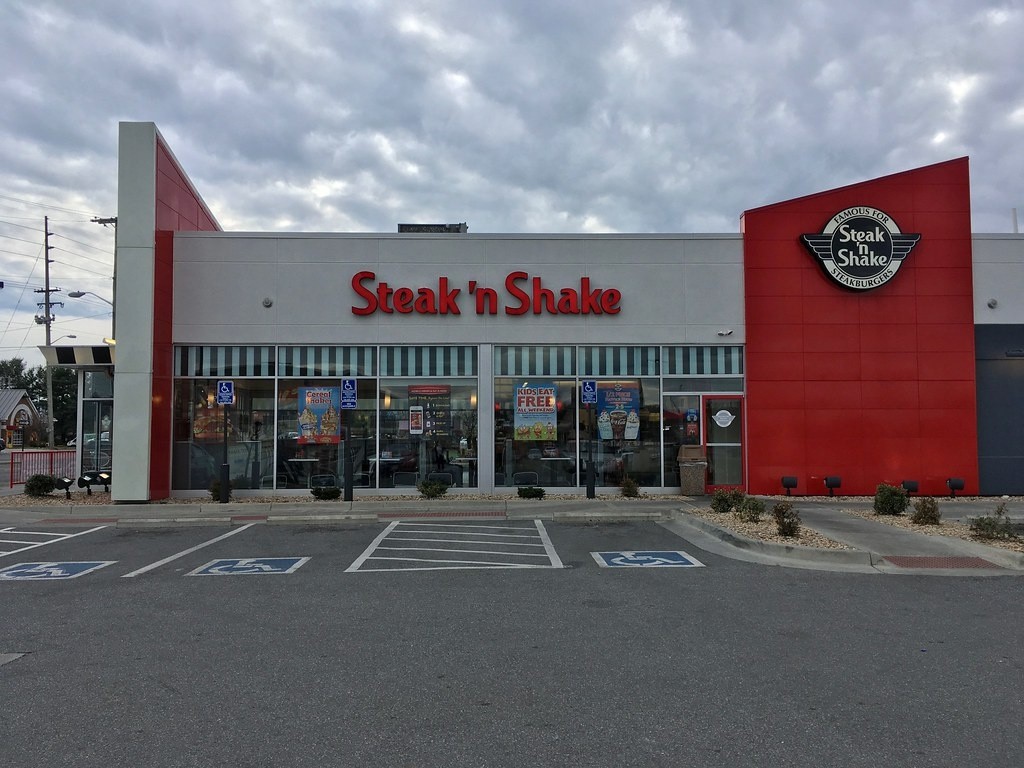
540;457;570;487
456;458;478;487
287;458;320;489
369;457;401;488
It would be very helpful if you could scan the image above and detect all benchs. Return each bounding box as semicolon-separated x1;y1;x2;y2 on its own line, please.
494;438;538;486
310;439;345;489
393;440;452;486
572;439;603;487
260;439;287;488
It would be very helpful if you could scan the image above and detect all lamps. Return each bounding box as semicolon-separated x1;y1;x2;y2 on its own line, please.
825;476;841;497
55;476;74;499
946;478;965;498
781;476;797;496
78;474;94;496
902;481;919;498
96;472;110;492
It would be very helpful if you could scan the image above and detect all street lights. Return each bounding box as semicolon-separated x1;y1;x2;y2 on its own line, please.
67;291;116;340
46;335;76;452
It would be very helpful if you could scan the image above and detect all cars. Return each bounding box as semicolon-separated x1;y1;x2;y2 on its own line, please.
0;438;5;451
172;441;216;488
66;432;110;447
